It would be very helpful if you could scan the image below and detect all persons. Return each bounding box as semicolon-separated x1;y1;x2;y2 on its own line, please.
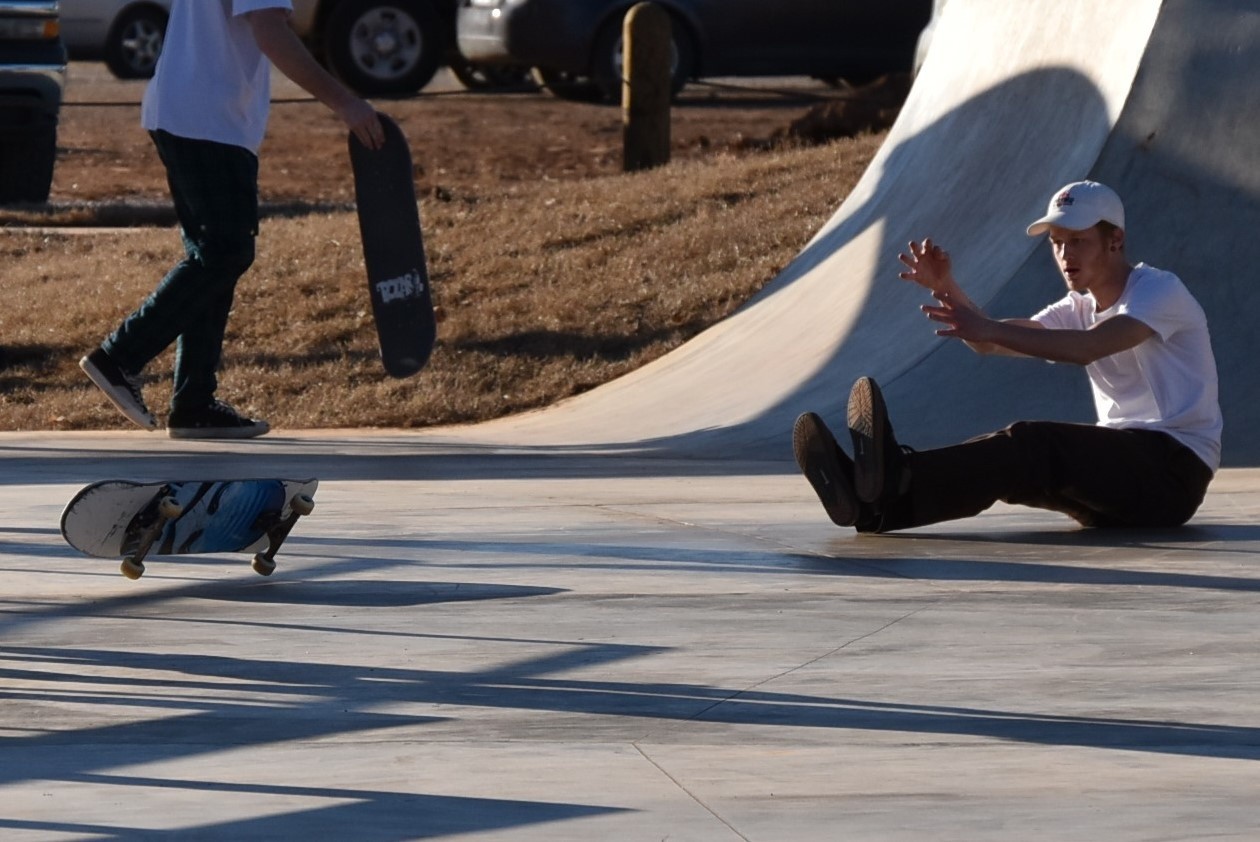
790;178;1224;536
75;0;390;446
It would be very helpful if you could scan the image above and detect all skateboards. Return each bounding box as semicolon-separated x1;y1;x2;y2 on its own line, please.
345;111;438;380
59;471;322;581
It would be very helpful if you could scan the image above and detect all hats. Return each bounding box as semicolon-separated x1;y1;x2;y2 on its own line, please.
1025;179;1126;233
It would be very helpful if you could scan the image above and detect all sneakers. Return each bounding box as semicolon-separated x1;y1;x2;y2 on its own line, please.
165;401;271;438
846;374;917;499
80;348;155;430
794;412;866;526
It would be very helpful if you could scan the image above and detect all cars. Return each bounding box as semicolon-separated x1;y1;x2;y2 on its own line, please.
52;0;184;83
276;0;485;98
0;0;70;204
456;1;934;102
